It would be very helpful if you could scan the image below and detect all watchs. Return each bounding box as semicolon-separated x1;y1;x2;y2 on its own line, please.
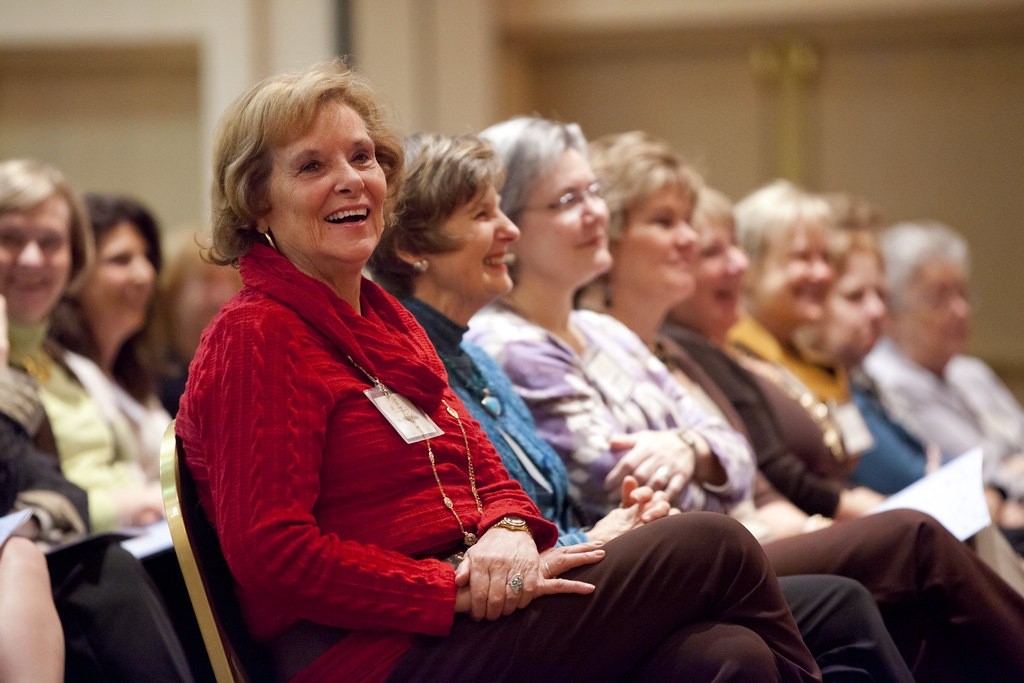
489;517;534;535
674;426;699;465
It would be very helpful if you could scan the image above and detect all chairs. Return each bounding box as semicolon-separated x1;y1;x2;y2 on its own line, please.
155;409;261;683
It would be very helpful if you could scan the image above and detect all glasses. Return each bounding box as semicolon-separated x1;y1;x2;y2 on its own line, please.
510;183;604;219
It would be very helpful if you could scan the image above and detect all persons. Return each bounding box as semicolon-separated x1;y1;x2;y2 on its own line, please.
175;68;824;683
468;115;1024;683
590;128;833;537
0;155;237;682
667;178;1024;593
365;131;915;682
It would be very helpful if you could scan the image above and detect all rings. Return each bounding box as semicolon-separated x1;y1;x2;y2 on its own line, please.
507;574;524;593
545;563;551;575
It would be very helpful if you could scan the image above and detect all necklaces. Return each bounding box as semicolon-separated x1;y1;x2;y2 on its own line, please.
344;352;484;547
439;351;500;418
713;339;841;453
